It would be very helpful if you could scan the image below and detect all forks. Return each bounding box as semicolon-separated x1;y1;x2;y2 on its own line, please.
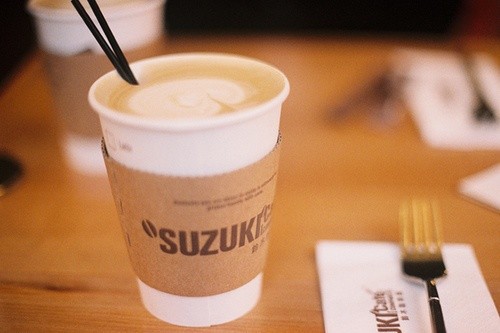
397;194;450;333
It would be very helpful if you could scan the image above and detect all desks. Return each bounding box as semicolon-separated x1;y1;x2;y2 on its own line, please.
0;33;500;333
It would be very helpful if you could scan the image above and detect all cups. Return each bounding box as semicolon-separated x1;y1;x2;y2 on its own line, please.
28;1;169;180
87;52;291;327
